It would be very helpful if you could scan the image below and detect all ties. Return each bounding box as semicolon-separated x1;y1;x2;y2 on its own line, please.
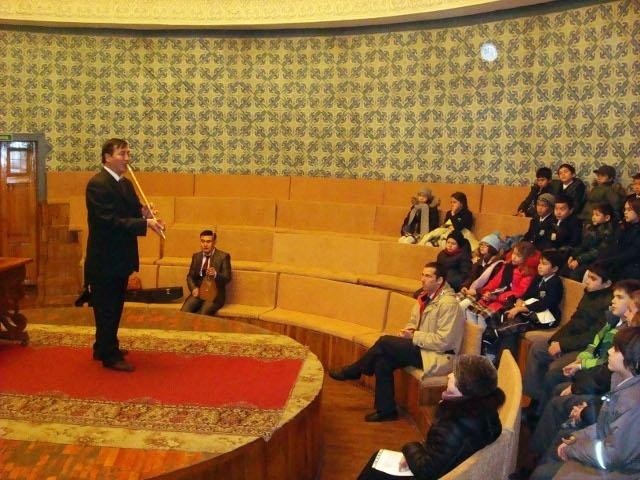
202;255;210;278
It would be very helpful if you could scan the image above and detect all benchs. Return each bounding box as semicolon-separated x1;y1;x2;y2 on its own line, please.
45;169;640;479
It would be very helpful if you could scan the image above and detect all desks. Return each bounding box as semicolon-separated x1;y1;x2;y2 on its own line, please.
1;256;33;348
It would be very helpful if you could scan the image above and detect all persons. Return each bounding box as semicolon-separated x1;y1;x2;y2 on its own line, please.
358;354;505;479
330;262;465;423
399;162;639;478
180;229;232;317
83;138;165;371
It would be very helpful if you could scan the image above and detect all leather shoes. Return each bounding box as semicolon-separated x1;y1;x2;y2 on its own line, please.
365;408;399;422
102;358;136;371
94;348;127;361
328;365;362;381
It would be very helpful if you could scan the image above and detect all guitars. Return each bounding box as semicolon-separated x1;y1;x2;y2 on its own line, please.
199;225;218;301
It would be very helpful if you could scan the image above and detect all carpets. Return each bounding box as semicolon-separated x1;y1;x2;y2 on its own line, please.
1;322;312;447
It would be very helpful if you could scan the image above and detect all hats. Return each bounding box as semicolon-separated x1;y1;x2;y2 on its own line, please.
480;232;500;252
417;187;432;200
446;229;465;247
593;165;616;177
537;192;555;208
